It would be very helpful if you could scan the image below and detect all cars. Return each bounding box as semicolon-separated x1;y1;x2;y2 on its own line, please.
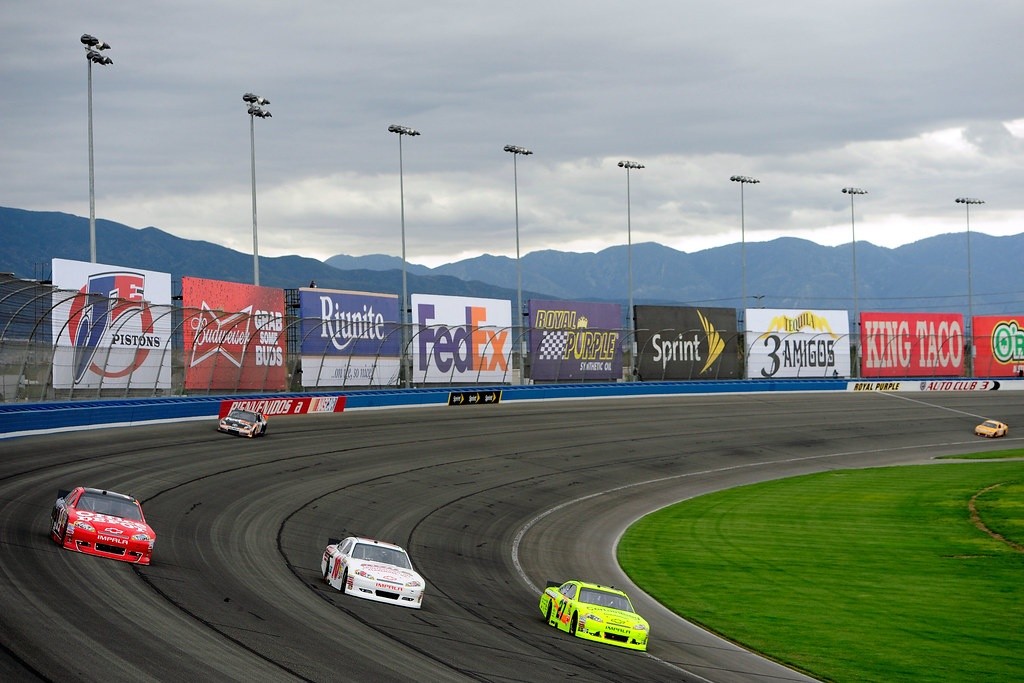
320;535;426;610
218;406;269;438
538;581;650;652
975;419;1009;438
47;484;156;566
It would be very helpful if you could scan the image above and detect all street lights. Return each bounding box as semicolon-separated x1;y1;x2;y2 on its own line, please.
81;31;113;262
730;174;760;311
388;123;422;388
842;185;870;381
956;196;986;315
243;90;272;286
616;159;646;381
503;143;534;383
